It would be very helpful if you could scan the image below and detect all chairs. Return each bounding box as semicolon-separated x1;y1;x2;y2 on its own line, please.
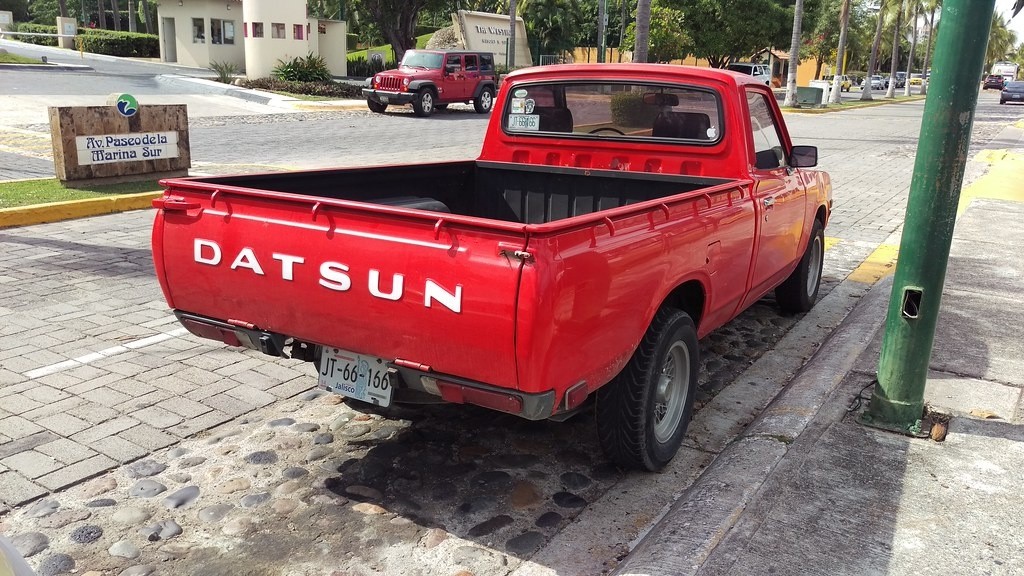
652;112;710;139
525;106;574;131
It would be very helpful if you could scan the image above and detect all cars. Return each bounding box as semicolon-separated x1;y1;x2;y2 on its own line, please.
885;69;931;87
999;81;1024;104
860;74;886;90
828;74;852;92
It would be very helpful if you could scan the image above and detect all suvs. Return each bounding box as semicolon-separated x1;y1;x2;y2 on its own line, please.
982;74;1007;90
361;48;500;119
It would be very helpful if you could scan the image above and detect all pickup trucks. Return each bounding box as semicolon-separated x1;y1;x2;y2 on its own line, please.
147;62;835;475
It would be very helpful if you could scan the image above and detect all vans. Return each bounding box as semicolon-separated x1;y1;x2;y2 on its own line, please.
726;63;772;87
